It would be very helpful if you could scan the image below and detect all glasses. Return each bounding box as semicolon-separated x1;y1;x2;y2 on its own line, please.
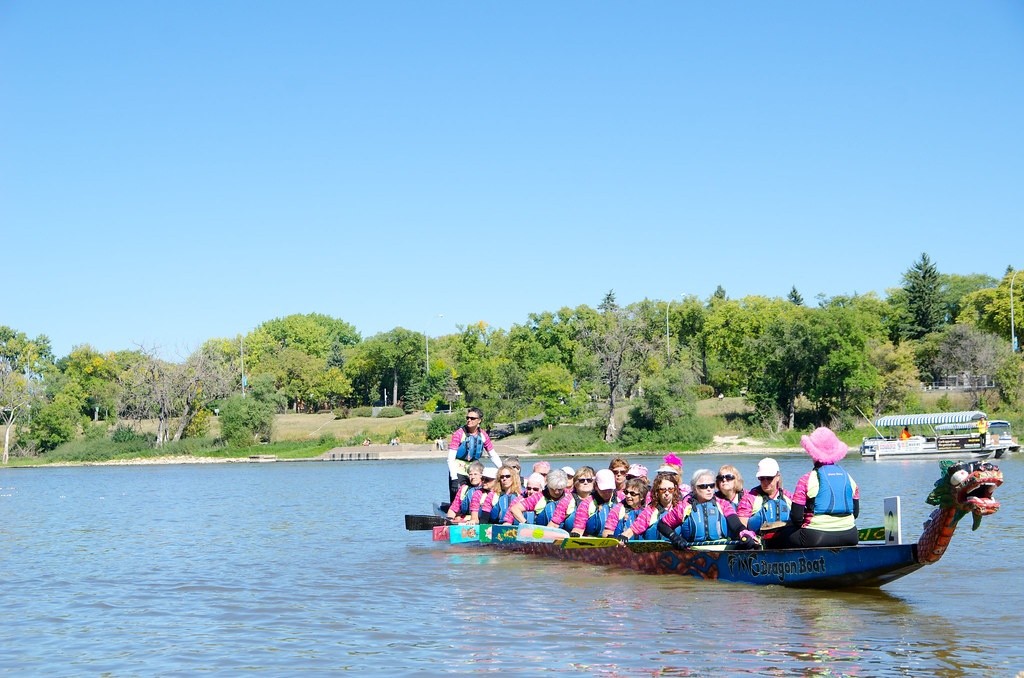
527;487;540;492
512;466;519;470
500;475;512;479
657;488;675;494
578;478;594;483
758;477;773;481
716;474;736;480
613;470;626;475
541;473;547;477
626;476;635;480
697;483;715;489
466;416;479;420
623;490;640;496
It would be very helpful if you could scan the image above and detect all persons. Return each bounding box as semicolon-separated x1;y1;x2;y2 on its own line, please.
362;437;371;446
481;456;596;533
434;436;448;450
446;460;484;524
767;426;859;550
901;426;911;438
569;453;692;544
657;457;794;550
461;467;499;525
976;415;989;448
387;436;400;445
446;406;502;506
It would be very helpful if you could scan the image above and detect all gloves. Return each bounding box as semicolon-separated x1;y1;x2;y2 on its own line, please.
451;479;458;493
570;532;581;537
617;535;628;543
739;534;754;548
670;532;690;550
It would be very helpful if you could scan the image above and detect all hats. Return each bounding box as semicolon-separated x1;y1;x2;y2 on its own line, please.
626;464;648;479
563;467;575;477
756;458;779;477
656;464;678;475
801;427;848;464
596;469;616;490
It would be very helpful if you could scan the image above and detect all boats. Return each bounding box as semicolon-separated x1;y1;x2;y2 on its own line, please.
404;458;1005;592
860;411;1022;462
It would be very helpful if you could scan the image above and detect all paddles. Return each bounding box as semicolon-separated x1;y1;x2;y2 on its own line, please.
404;512;886;552
